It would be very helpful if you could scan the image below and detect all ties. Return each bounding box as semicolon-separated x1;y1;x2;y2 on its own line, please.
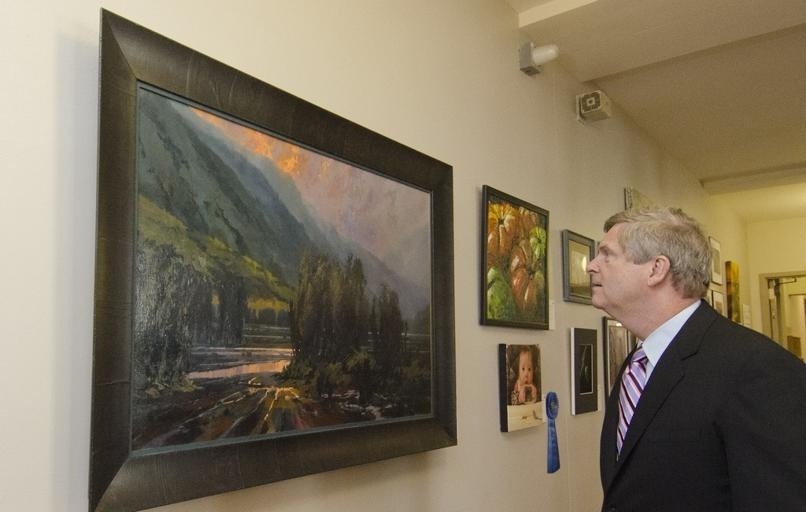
616;347;647;459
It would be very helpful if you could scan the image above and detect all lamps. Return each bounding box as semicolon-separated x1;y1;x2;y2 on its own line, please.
519;40;560;76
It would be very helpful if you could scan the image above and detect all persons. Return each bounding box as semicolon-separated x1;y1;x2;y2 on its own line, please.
510;348;539;406
585;205;805;512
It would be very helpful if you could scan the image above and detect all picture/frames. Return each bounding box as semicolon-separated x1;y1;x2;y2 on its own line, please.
88;7;458;511
479;183;629;431
704;235;726;318
